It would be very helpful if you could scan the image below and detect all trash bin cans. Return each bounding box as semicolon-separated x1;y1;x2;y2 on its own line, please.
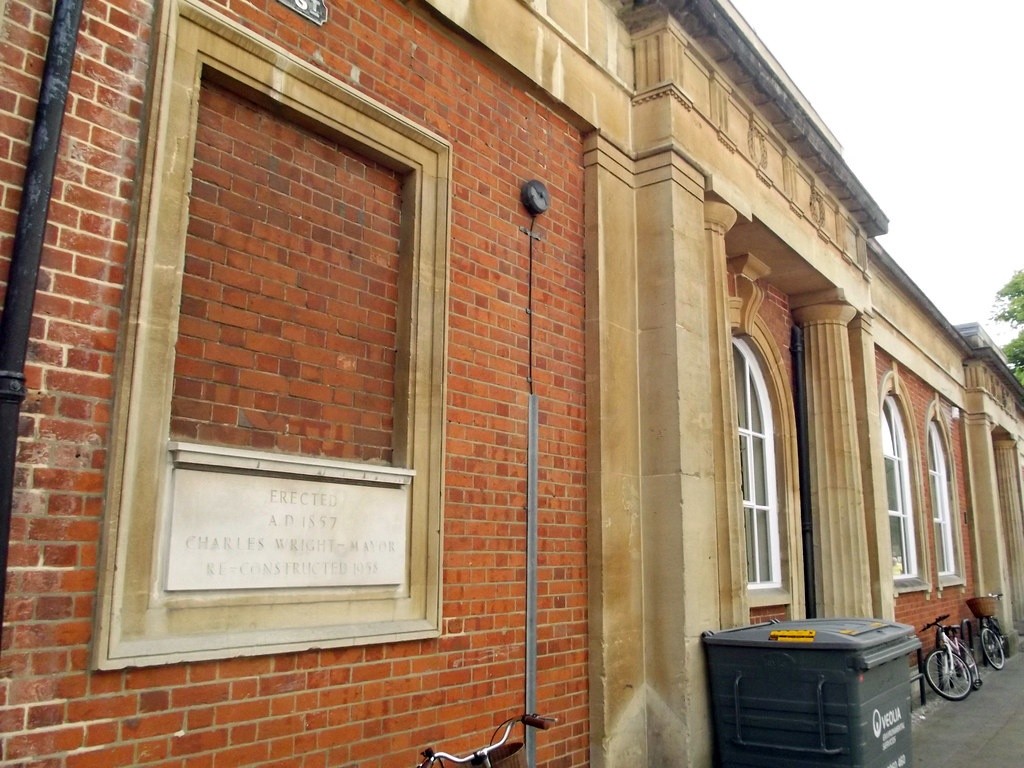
699;617;924;768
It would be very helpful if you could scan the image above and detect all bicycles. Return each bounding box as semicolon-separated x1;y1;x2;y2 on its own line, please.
416;710;558;768
965;593;1010;670
918;614;984;701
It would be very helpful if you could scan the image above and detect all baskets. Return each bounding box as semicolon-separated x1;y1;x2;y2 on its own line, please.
965;597;996;618
